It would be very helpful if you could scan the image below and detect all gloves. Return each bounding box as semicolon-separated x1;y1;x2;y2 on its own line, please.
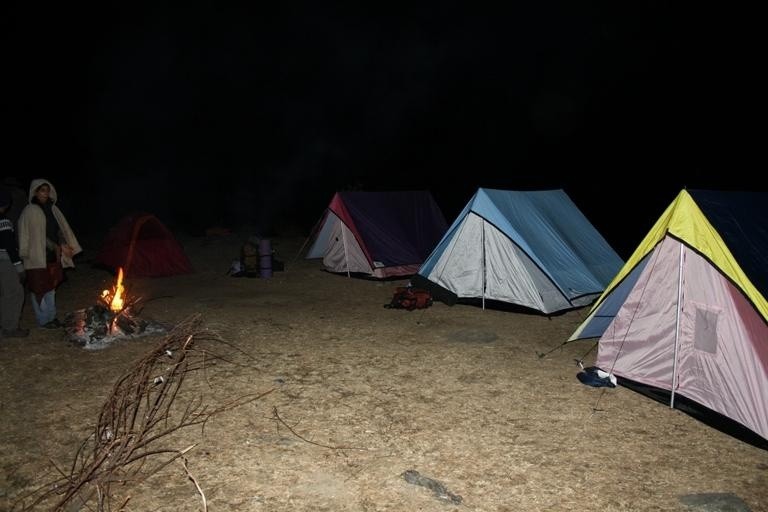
18;264;27;283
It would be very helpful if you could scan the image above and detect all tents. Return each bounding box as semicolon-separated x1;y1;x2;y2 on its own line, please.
298;188;449;279
562;184;768;444
408;186;626;314
90;211;196;277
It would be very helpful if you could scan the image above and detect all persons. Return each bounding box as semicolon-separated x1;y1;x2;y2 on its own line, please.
0;188;30;337
18;178;84;328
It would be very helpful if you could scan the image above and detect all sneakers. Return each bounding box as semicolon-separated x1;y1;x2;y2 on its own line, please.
4;318;63;337
